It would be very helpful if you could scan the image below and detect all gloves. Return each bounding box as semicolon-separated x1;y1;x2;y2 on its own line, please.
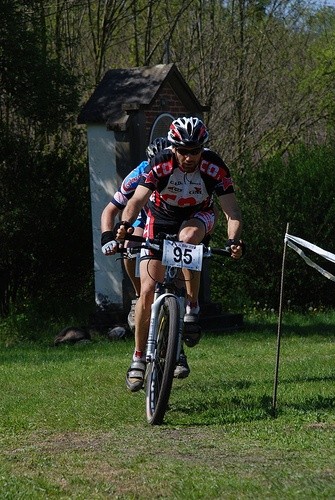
101;240;117;255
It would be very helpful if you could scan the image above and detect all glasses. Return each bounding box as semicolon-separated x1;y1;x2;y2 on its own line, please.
176;147;201;155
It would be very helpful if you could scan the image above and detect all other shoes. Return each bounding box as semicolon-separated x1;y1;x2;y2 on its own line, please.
173;355;191;379
126;299;139;329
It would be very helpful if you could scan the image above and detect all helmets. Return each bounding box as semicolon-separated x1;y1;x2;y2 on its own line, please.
167;116;210;150
145;137;172;160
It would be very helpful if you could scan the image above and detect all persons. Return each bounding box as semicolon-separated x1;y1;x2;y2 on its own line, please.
101;136;190;378
114;117;242;390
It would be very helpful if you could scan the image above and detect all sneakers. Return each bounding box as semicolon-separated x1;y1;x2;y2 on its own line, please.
125;351;147;392
182;304;203;347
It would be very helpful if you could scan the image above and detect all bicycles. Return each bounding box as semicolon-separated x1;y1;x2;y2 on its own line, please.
115;223;239;426
104;241;181;409
